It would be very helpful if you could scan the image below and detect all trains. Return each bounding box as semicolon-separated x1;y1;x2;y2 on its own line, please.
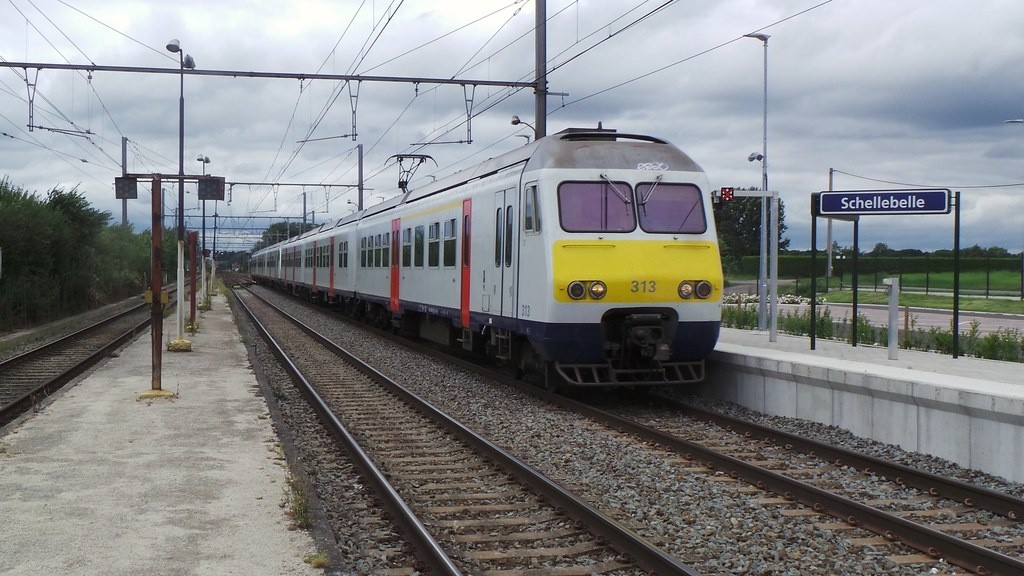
249;117;725;401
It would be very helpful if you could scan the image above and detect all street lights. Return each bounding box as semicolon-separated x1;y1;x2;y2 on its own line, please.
742;32;772;332
196;153;212;311
165;38;192;353
